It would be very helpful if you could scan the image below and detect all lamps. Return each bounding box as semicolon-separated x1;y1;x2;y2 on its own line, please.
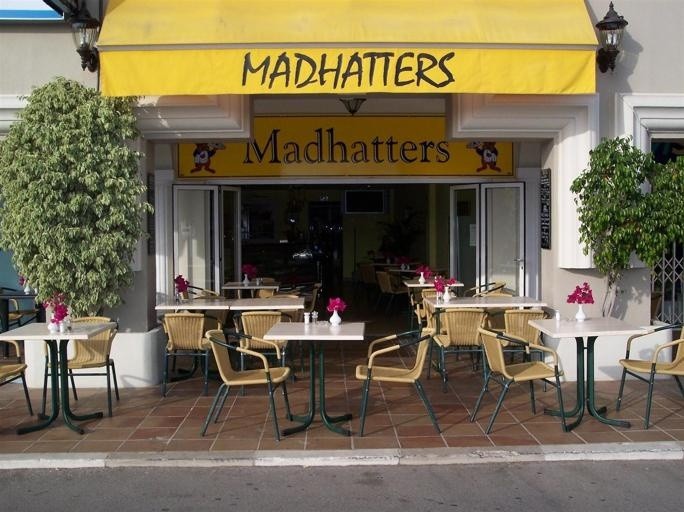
61;0;98;73
337;96;369;115
593;0;628;76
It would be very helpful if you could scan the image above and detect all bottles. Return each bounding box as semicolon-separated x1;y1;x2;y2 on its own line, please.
407;265;410;270
304;312;310;325
66;318;73;334
175;293;181;303
255;276;264;285
312;310;319;325
59;320;65;333
555;310;561;321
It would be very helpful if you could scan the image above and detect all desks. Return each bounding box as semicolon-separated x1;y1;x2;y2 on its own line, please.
1;321;116;435
527;316;648;430
155;297;305;333
265;321;365;438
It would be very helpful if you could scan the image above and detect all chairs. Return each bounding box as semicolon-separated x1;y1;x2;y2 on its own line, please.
0;340;34;418
617;325;683;429
355;327;442;436
159;312;292;444
177;278;323;311
424;282;568;436
41;316;120;418
379;266;459;327
2;286;22;329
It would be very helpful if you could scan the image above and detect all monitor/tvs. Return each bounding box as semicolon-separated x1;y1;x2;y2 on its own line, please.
344;190;384;215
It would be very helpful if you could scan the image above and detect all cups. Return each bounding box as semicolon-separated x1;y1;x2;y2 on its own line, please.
437;292;443;302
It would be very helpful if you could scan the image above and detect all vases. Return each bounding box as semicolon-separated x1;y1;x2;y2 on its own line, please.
574;305;587;322
330;313;342;328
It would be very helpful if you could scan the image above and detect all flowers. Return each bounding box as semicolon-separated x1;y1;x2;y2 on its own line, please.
566;282;595;304
328;298;346;312
